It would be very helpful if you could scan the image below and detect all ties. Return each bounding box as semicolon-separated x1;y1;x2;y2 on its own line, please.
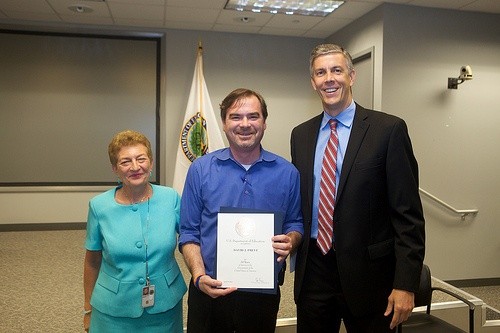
317;119;338;255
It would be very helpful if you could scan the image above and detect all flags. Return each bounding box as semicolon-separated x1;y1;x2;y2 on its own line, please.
173;56;226;196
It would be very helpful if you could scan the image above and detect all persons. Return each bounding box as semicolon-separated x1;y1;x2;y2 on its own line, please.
144;288;148;295
290;44;426;333
82;130;188;333
178;88;305;333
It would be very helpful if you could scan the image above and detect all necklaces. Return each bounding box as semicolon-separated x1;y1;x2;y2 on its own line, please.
141;187;150;202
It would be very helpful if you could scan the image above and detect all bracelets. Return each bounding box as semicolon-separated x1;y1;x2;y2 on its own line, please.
84;309;91;314
193;274;204;289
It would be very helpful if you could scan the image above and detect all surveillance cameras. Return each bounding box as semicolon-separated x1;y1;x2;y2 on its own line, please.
460;64;471;77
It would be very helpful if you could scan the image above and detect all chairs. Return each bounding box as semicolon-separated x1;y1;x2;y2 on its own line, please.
395;264;476;333
